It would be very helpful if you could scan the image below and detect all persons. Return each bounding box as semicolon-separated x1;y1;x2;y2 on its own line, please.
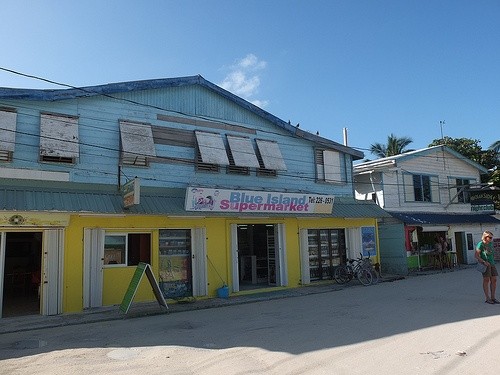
433;236;450;271
474;231;500;304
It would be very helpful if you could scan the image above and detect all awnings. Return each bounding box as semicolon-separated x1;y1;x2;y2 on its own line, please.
390;212;500;224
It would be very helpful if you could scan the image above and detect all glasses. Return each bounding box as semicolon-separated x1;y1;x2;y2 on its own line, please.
487;236;493;239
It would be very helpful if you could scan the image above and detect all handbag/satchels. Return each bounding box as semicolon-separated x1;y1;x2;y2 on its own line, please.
477;260;488;274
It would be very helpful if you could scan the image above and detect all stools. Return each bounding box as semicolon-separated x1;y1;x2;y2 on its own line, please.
433;252;460;271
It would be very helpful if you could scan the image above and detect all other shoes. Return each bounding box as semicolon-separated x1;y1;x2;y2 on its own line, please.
492;299;500;304
484;299;495;304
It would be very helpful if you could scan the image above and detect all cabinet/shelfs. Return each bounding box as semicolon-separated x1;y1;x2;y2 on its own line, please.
266;229;276;285
308;233;340;281
159;237;191;248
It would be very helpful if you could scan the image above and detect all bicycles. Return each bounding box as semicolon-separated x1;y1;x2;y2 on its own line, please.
333;252;381;286
